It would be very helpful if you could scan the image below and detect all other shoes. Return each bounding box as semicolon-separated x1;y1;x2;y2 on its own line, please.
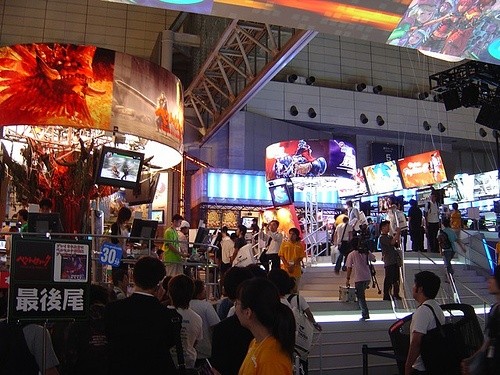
342;265;347;272
393;296;401;300
383;296;391;301
335;266;341;271
360;316;369;322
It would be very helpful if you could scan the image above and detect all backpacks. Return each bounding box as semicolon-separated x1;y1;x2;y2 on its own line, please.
0;320;39;375
438;229;451;249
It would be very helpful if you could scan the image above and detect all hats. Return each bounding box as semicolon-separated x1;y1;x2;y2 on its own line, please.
180;221;190;228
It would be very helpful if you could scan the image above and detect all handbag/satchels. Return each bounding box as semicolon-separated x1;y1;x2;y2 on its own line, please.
330;245;340;264
394;209;409;232
340;224;353;253
351;209;368;231
194;358;213;375
99;223;119;247
395;242;403;267
292;308;321;357
418;304;470;375
169;309;193;375
367;250;376;276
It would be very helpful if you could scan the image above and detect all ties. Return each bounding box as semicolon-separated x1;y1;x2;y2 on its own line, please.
261;237;272;257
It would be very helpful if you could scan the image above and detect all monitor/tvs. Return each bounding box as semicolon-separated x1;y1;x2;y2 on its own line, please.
269;183;295;207
28;212;59;233
94;146;145;189
129;219;158;244
242;216;258;230
193;227;209;249
336;150;448;212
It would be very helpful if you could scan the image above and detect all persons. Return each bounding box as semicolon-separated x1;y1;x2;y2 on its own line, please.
405;271;446;375
121;169;128;180
0;198;323;375
334;140;356;179
431;151;444;181
460;264;500;375
333;189;500;321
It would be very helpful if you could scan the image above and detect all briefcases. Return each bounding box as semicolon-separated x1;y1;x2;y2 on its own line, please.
339;284;356;303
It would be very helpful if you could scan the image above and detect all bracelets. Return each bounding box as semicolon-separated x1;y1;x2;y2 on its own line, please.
346;277;350;280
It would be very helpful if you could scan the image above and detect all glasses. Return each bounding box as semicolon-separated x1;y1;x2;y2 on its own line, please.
234;301;241;308
